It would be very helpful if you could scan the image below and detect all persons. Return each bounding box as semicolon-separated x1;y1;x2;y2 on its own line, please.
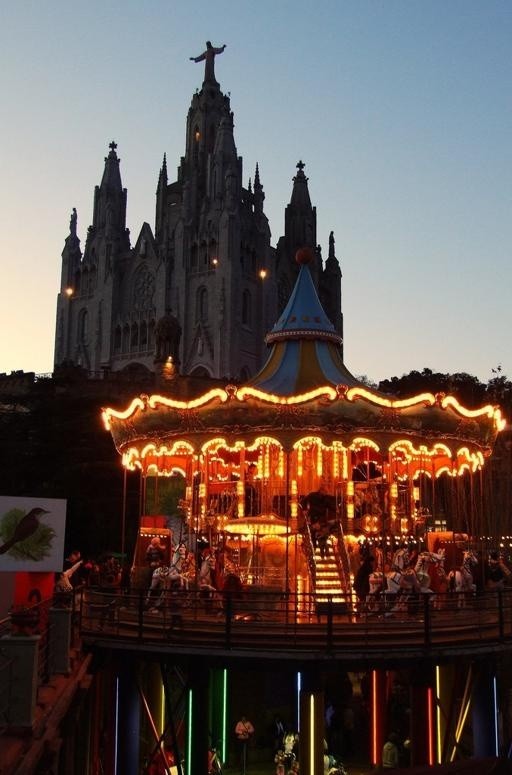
235;716;254;768
54;538;124;615
351;546;511;619
304;504;331;560
